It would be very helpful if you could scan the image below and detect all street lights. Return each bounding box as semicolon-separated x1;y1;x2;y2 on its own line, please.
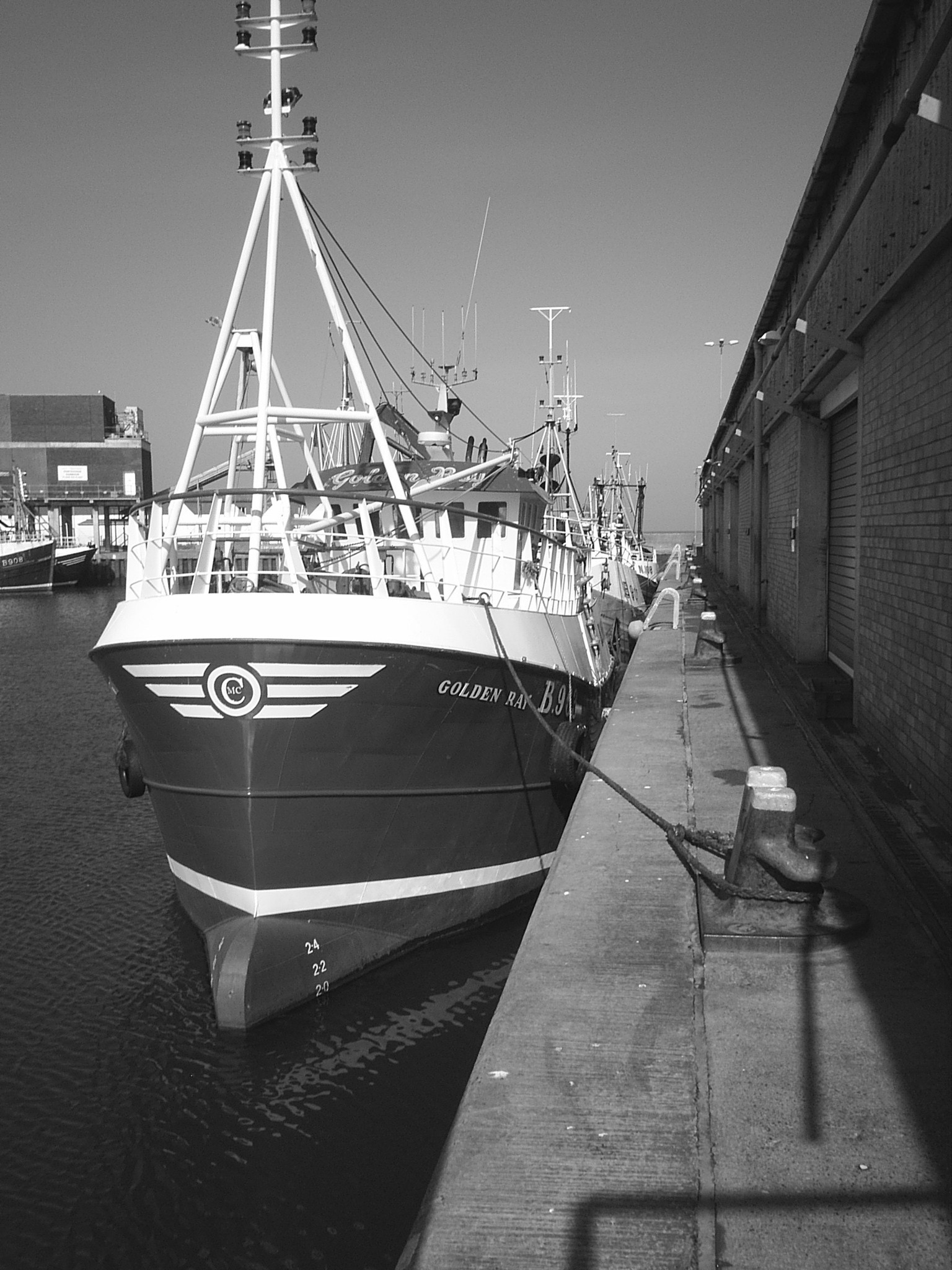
704;338;742;412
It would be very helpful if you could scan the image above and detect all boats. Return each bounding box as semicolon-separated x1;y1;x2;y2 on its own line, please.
0;466;56;594
55;544;98;591
88;1;660;1036
